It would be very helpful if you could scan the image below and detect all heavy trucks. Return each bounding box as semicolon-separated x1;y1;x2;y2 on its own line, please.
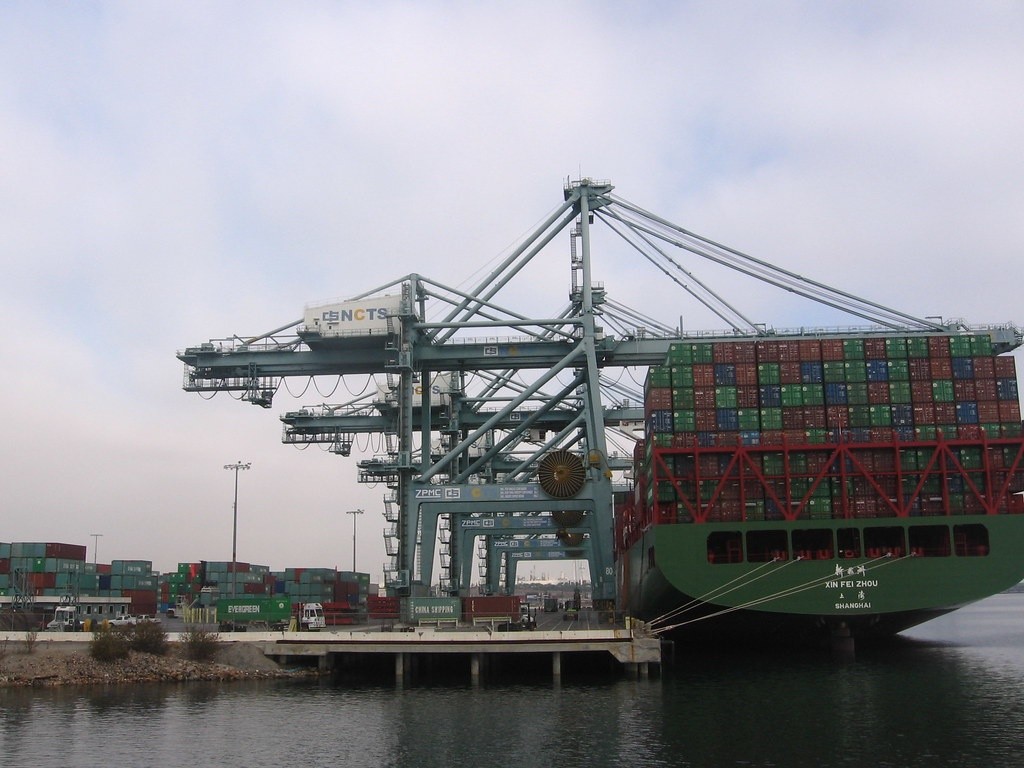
216;598;326;631
398;597;537;632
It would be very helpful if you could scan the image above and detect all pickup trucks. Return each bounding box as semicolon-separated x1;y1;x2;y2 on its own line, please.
108;615;136;627
136;615;162;624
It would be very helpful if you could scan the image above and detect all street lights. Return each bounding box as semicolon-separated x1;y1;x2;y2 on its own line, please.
90;533;104;563
346;509;365;601
224;460;252;598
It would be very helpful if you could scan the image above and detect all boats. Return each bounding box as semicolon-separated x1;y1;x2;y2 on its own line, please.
615;338;1024;650
